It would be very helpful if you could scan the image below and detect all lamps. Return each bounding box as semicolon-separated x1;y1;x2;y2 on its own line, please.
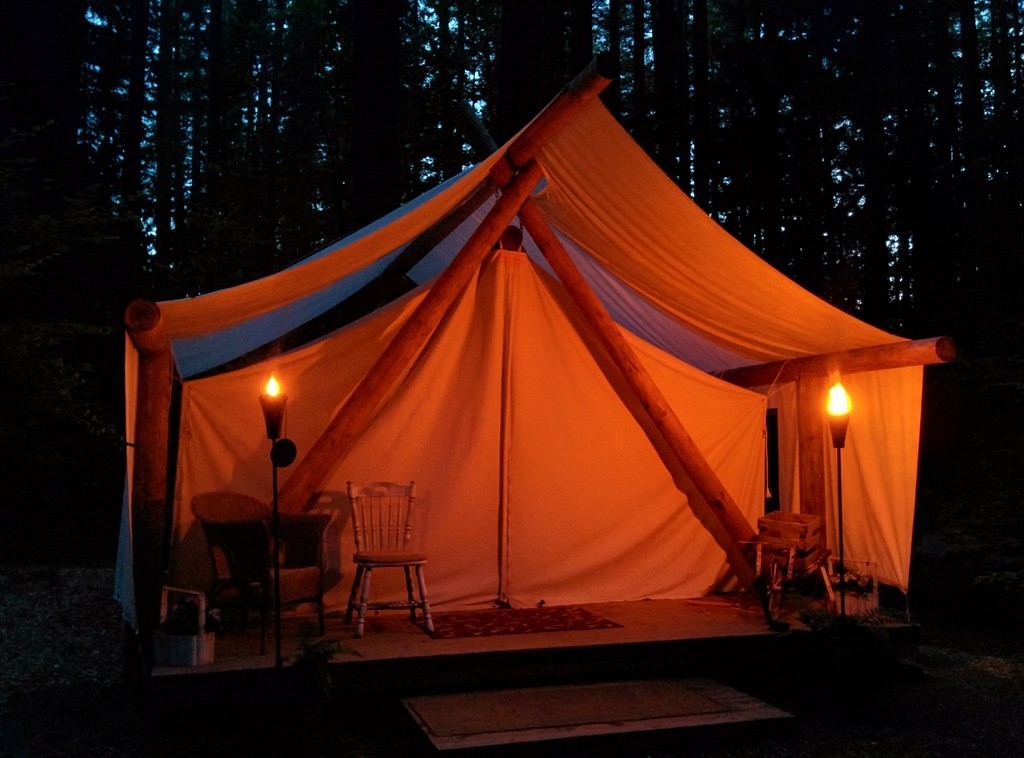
826;383;850;616
258;368;289;666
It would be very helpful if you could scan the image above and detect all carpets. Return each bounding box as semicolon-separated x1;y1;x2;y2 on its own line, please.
407;680;733;738
407;605;624;639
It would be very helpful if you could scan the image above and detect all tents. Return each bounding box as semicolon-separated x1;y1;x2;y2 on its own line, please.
124;58;959;696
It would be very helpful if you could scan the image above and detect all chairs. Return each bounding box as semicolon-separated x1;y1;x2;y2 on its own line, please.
190;492;332;656
343;480;435;639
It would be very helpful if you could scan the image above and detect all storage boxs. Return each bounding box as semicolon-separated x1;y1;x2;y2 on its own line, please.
758;510;820;579
